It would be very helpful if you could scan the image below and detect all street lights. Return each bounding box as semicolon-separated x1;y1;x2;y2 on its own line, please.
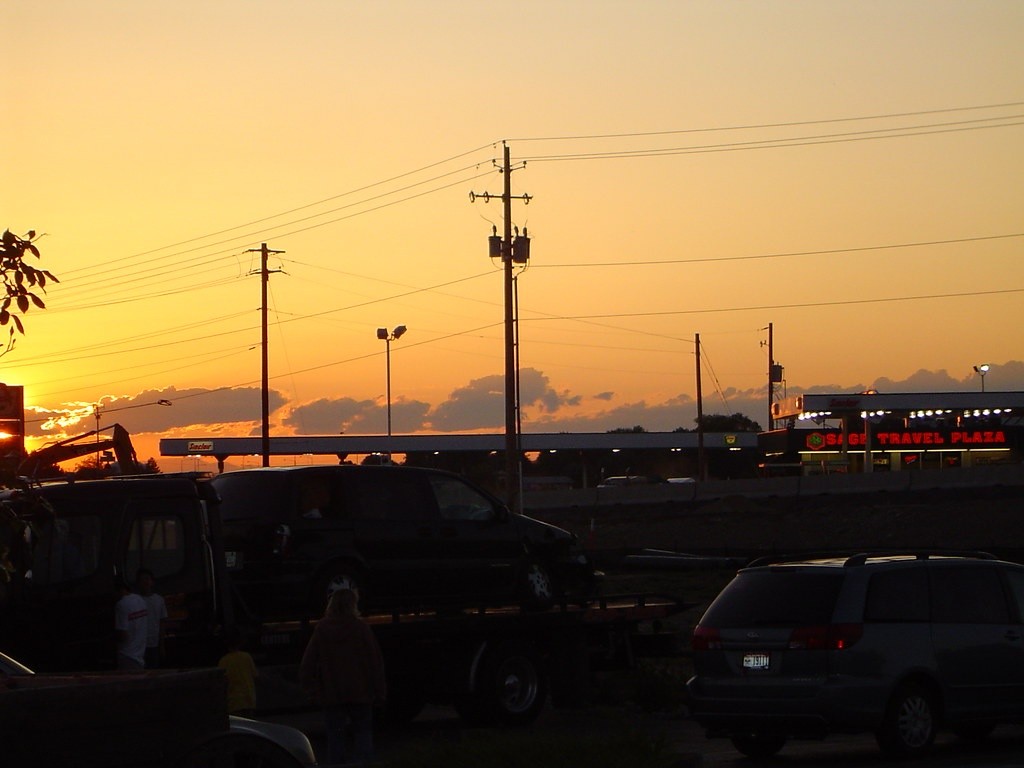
376;325;409;464
972;364;990;392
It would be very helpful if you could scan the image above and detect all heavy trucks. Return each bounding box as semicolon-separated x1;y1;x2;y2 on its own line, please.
0;466;674;741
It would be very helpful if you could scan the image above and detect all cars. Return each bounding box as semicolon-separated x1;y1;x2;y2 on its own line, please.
0;647;321;768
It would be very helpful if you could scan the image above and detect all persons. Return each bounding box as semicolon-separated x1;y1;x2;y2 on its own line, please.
113;569;168;670
299;589;385;764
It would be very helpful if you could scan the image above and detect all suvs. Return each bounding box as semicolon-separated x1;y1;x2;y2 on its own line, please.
206;453;604;612
683;546;1024;763
